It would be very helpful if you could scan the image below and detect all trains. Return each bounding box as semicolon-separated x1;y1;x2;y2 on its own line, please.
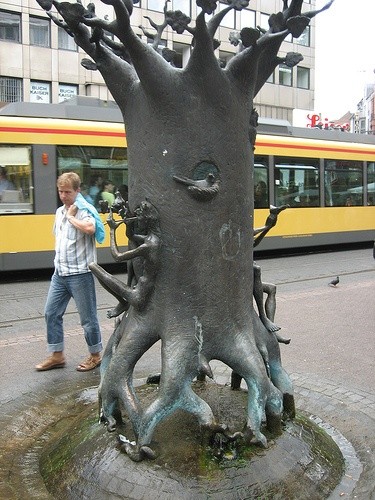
0;93;375;276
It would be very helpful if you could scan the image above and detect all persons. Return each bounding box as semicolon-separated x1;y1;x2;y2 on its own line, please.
253;180;269;207
345;182;370;207
89;200;162;319
275;187;291;207
36;172;104;372
115;184;129;205
254;203;293;346
0;165;14;198
298;188;319;206
89;173;104;199
80;183;95;206
94;179;115;210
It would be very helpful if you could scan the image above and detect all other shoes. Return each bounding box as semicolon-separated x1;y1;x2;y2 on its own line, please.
34;355;66;371
76;352;103;372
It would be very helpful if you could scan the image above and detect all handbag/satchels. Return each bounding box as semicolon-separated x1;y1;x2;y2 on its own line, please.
73;192;105;245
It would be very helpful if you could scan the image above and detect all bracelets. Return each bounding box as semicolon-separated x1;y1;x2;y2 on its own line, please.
67;215;74;222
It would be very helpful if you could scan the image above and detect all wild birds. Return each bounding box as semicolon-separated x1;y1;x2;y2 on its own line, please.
328;277;339;287
173;173;214;187
113;191;125;205
